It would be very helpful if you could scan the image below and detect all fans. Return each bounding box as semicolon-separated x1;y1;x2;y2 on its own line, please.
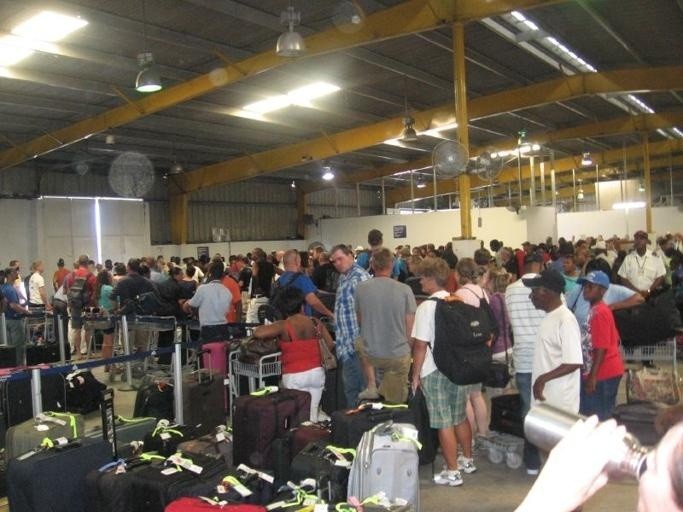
471;150;504;181
431;140;470;179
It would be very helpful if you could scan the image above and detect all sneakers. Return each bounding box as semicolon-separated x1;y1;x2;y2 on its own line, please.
433;431;490;488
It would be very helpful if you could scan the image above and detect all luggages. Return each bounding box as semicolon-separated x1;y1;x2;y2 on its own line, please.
488;393;524;438
0;342;436;512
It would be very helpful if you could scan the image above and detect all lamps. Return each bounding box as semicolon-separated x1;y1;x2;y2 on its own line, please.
415;171;428;191
134;1;164;94
276;0;305;58
168;139;183;175
399;79;418;142
321;162;336;181
582;137;593;166
577;180;585;200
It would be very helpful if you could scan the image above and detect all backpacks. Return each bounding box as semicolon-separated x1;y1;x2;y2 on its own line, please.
68;272;93;310
462;286;499;348
269;272;300;306
424;296;494;387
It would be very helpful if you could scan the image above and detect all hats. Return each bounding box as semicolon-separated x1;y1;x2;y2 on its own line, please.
522;270;565;294
576;270;609;289
591;240;607;250
635;230;651;244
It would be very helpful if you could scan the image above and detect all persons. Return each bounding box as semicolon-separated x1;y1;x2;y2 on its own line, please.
2;229;682;488
513;404;682;512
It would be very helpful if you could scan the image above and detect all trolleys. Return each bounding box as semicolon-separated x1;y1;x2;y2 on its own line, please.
611;335;683;449
79;306;199;383
474;428;527;471
24;306;54;345
226;349;283;428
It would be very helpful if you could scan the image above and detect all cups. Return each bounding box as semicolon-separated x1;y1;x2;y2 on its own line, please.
523;403;646;482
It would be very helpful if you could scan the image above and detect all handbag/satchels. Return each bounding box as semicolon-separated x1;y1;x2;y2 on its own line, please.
483;363;510;389
626;368;677;406
136;292;165;315
614;286;683;347
